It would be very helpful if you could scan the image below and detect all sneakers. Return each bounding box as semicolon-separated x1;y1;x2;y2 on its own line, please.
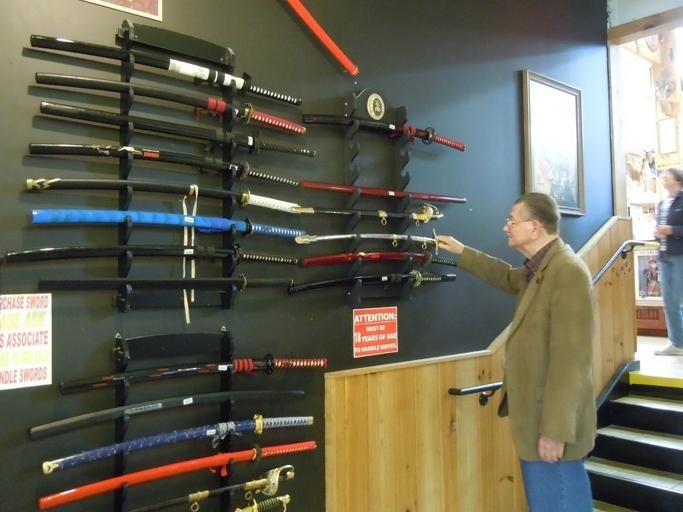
655;345;683;355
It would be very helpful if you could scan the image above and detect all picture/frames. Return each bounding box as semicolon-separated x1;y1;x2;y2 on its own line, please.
657;117;679;156
522;68;586;216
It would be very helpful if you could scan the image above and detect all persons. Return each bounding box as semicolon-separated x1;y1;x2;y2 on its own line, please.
652;168;683;356
435;193;598;511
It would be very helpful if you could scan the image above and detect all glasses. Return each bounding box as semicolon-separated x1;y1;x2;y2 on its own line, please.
506;217;533;226
